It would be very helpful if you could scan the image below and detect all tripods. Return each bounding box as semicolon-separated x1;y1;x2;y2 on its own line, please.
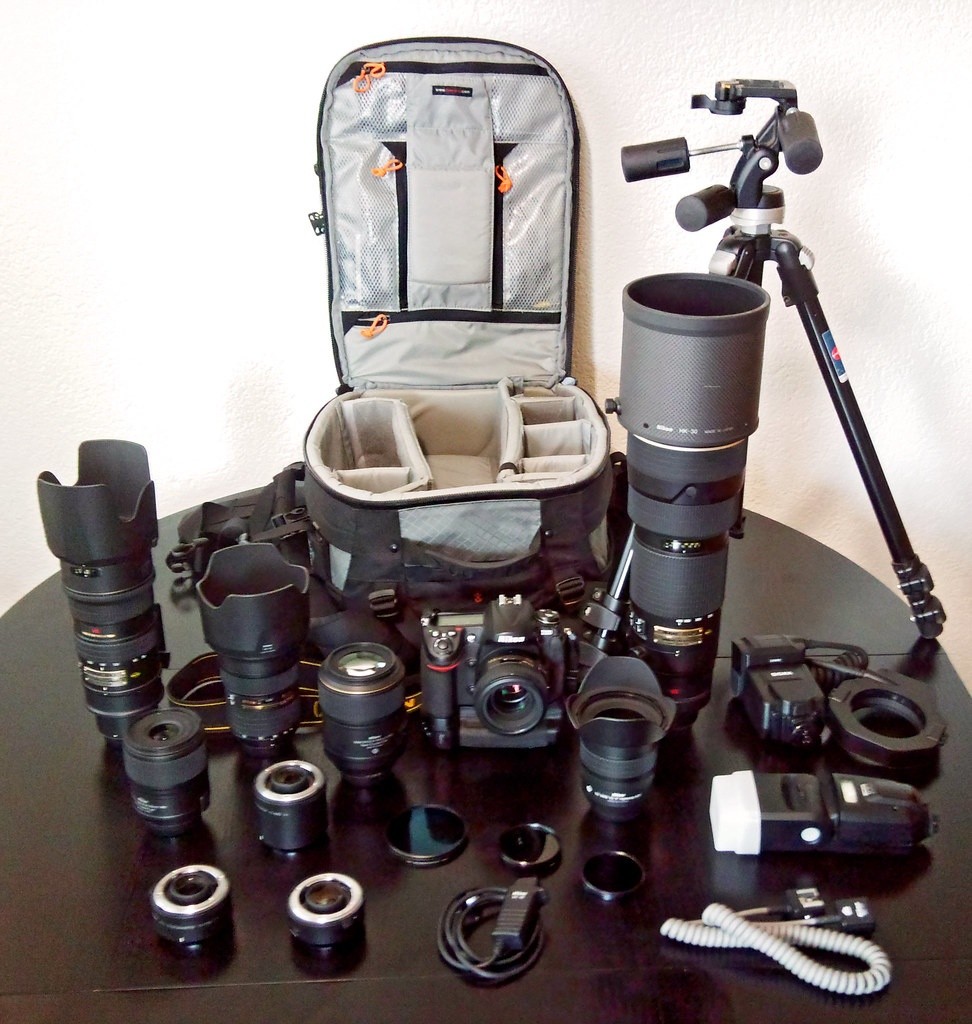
574;77;947;693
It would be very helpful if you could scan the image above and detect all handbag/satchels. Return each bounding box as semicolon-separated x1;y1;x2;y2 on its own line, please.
307;35;613;620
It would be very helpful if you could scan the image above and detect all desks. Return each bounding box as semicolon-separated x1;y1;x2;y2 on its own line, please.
0;465;972;1024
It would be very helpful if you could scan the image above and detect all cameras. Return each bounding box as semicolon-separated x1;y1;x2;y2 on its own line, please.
420;594;570;749
